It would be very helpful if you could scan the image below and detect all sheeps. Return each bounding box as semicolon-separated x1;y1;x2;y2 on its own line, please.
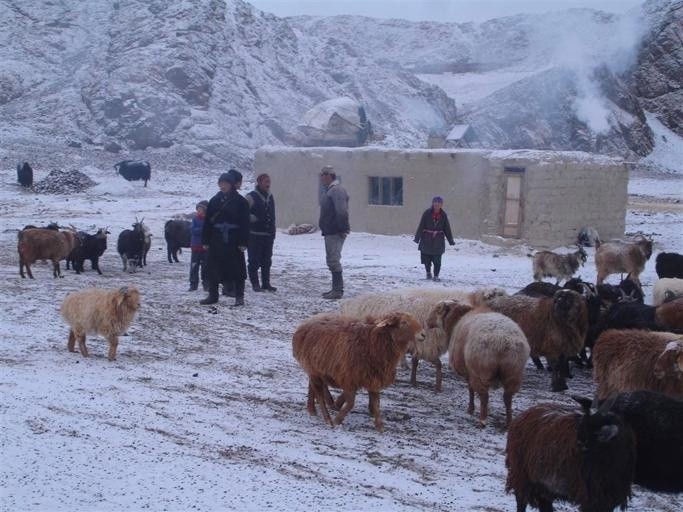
487;224;683;512
17;216;153;279
17;162;33;188
164;219;192;263
289;282;530;434
114;159;151;187
60;284;144;362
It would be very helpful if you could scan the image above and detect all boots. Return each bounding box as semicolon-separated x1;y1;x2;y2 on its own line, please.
323;272;343;299
262;279;277;292
253;280;269;292
236;288;244;305
200;287;219;304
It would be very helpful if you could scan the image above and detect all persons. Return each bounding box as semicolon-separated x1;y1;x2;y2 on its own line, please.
319;165;350;299
244;174;277;292
189;169;250;310
412;197;455;281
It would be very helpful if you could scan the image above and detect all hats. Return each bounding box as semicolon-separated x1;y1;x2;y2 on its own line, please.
218;173;235;185
321;165;336;175
196;200;209;212
228;170;242;182
